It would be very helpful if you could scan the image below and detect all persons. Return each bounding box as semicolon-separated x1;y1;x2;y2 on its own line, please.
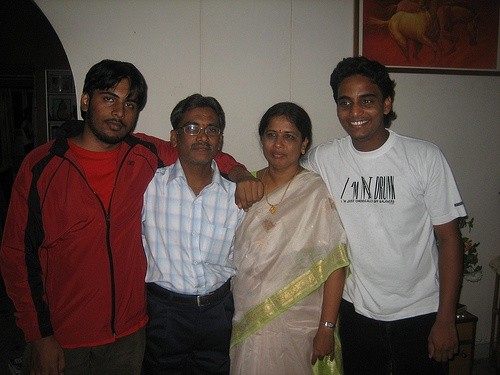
0;59;264;375
299;56;469;375
229;102;351;375
141;93;249;375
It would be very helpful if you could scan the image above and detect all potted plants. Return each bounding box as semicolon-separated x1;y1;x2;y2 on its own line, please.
457;216;483;315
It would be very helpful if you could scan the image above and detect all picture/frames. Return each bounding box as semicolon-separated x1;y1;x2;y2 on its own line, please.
353;1;500;76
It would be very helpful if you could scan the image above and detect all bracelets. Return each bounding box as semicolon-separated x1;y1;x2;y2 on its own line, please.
318;321;335;328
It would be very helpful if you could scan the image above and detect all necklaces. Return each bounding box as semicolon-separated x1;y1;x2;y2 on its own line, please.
263;164;301;214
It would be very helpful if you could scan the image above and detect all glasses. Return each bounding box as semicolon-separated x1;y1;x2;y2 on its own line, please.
174;123;223;138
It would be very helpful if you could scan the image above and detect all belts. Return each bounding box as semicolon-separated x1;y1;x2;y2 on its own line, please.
146;279;230;306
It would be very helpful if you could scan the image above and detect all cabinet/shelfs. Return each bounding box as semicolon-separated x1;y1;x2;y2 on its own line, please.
448;310;479;375
